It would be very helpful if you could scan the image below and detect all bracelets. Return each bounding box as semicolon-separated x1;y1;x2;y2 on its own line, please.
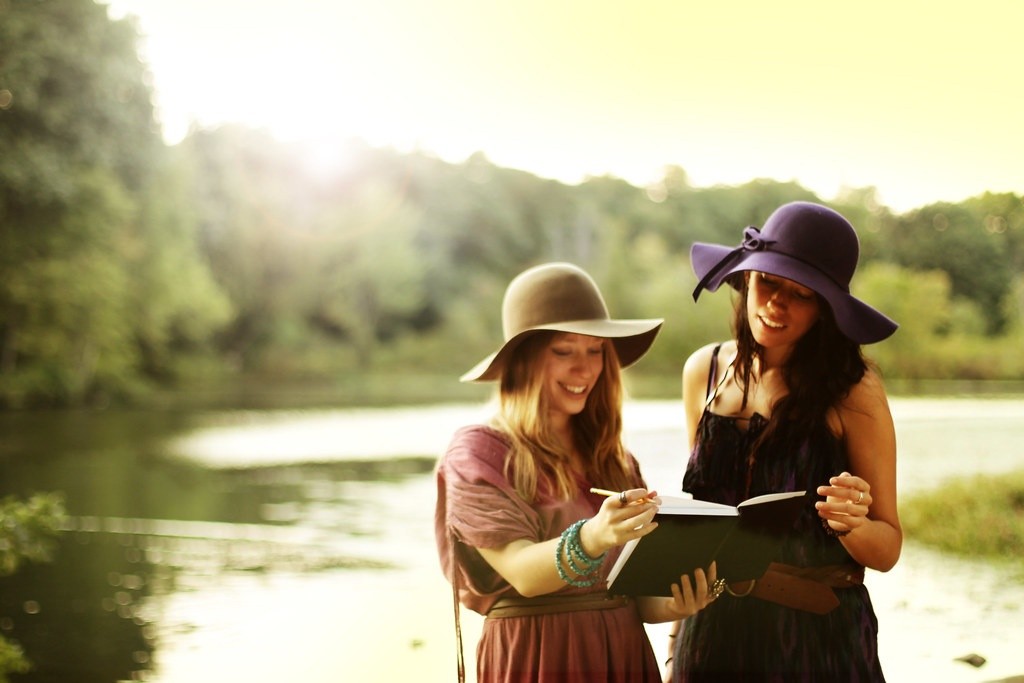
820;518;852;536
555;519;607;587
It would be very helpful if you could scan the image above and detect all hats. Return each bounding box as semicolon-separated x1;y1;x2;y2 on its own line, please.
457;263;663;381
689;201;897;345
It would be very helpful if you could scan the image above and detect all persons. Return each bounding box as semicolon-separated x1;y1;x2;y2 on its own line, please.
664;202;901;682
435;261;724;683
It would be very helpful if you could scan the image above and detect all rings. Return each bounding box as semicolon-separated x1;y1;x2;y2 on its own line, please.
618;492;627;506
708;578;725;598
855;492;864;503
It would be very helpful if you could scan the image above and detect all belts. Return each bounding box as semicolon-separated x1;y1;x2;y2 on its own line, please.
721;560;865;616
486;591;628;619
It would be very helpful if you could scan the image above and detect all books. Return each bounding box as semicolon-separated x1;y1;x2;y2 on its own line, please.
606;491;807;595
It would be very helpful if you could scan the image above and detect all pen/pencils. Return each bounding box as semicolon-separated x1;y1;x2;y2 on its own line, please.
588;486;659;507
